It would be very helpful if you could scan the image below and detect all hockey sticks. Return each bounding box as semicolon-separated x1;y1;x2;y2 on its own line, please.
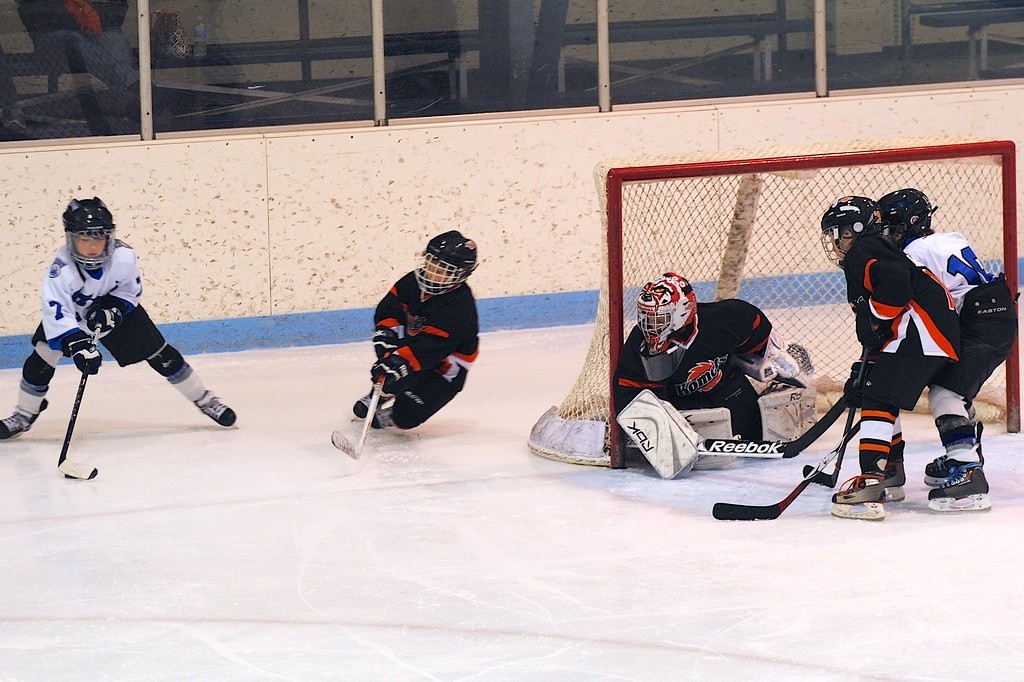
332;351;393;460
712;420;862;521
802;345;872;488
55;321;106;480
625;394;848;460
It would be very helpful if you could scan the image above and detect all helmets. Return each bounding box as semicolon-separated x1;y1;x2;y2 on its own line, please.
413;230;480;296
819;195;884;269
875;188;939;249
62;196;117;271
635;272;699;359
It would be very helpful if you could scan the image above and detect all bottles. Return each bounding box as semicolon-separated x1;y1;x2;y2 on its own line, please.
193;16;207;57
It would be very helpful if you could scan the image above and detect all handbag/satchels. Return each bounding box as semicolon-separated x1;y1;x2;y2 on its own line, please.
149;8;195;61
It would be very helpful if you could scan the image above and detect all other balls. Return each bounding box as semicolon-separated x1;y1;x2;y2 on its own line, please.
65;473;77;479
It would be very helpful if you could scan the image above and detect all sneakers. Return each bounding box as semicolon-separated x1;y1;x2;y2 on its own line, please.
353;388;395;420
0;399;48;440
830;471;885;521
193;390;239;429
370;405;398;429
928;445;992;512
923;421;985;485
883;440;906;502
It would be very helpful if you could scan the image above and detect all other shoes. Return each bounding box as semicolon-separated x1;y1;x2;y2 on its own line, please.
0;102;28;136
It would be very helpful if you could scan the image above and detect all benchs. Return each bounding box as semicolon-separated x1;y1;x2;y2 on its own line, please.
909;0;1024;80
0;0;832;140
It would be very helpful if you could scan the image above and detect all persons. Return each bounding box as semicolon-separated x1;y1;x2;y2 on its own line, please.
352;230;479;431
819;196;962;520
14;0;164;122
874;189;1019;513
614;272;814;480
0;197;237;442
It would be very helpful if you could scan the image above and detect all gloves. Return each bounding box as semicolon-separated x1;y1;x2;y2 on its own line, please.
855;302;895;355
61;330;102;375
373;330;403;359
85;292;128;333
371;354;413;386
843;359;875;409
